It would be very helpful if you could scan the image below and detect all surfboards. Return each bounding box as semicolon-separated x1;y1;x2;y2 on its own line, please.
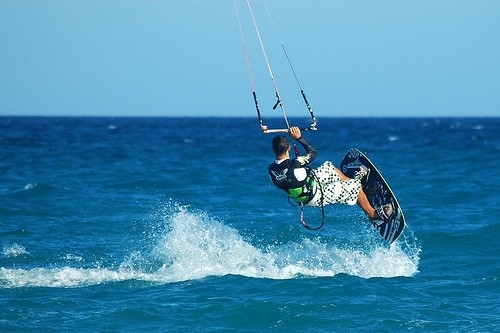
340;147;406;246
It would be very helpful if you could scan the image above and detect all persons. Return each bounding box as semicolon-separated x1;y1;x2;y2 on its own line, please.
268;126;393;221
